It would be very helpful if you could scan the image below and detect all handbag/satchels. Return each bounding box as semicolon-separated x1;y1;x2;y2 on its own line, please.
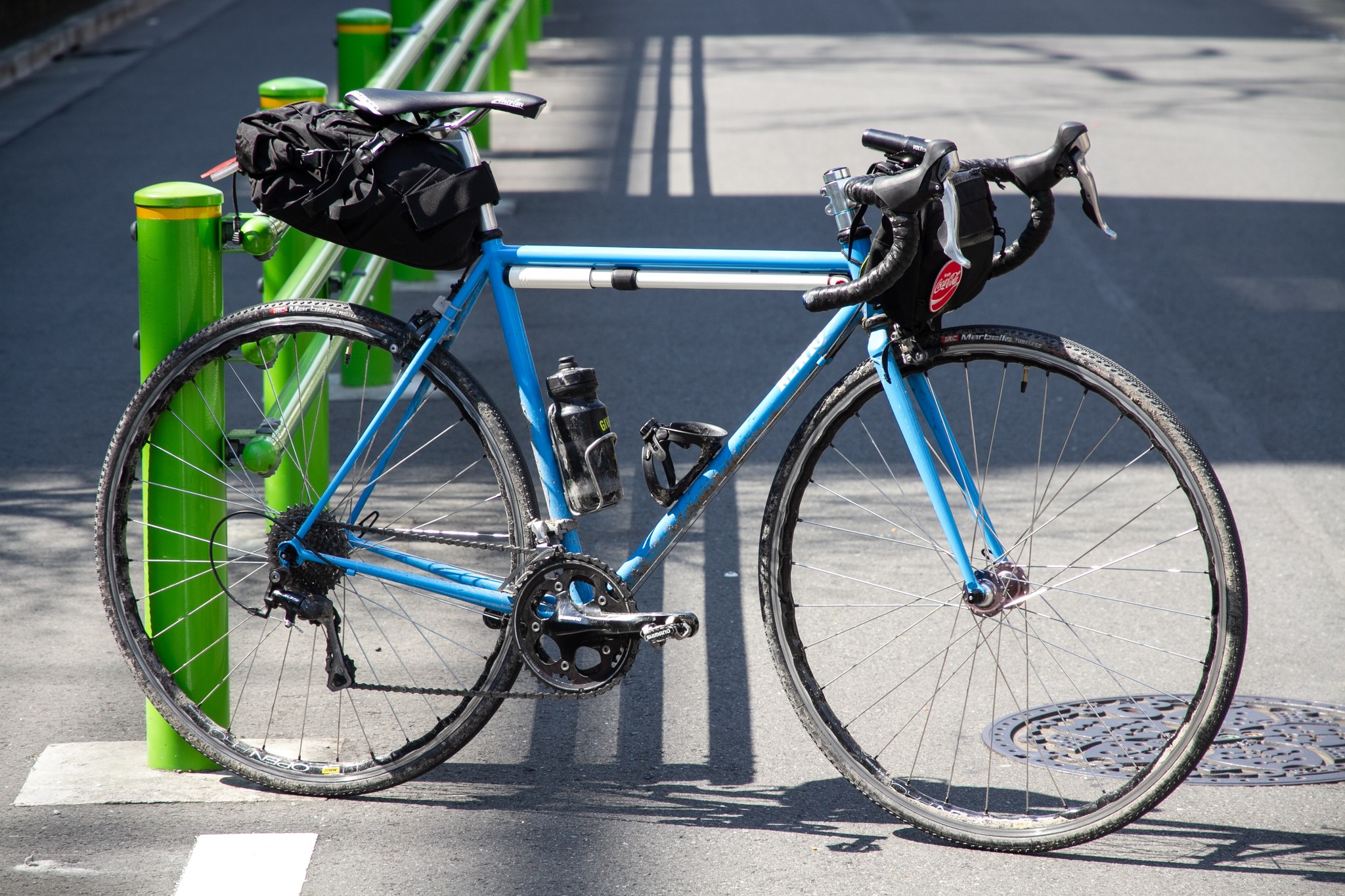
234;101;501;272
857;170;1005;326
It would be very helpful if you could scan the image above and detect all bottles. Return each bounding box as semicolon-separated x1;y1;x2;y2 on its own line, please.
547;354;623;514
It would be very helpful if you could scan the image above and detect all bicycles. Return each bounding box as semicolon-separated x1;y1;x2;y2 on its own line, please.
94;85;1250;854
